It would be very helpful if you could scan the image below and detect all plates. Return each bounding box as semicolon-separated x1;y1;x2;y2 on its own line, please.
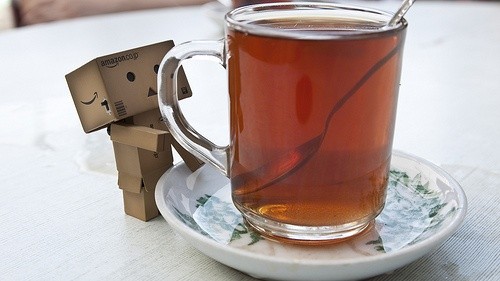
154;148;468;280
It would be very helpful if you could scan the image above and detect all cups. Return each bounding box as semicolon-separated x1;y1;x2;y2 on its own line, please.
156;1;409;246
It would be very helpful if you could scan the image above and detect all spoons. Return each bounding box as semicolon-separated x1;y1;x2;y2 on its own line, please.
232;0;416;196
